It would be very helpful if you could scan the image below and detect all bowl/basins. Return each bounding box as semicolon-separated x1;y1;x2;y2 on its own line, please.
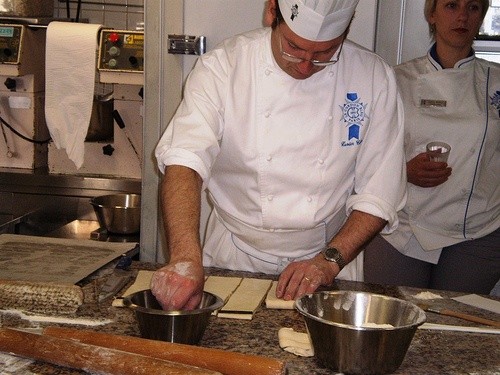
89;193;141;234
293;290;427;372
124;289;224;345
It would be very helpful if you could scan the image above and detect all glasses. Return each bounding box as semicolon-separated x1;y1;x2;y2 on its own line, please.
274;15;346;66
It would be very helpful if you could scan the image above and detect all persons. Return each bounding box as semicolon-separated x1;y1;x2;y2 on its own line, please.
389;0;500;295
149;0;409;313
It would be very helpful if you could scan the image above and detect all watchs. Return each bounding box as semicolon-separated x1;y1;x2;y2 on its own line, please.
319;247;346;271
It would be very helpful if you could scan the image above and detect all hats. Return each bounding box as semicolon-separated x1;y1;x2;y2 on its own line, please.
278;0;360;41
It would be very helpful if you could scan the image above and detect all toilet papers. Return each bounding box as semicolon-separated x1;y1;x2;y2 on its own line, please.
44;21;105;169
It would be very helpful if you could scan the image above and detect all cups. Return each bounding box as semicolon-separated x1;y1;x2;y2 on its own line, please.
427;142;451;170
